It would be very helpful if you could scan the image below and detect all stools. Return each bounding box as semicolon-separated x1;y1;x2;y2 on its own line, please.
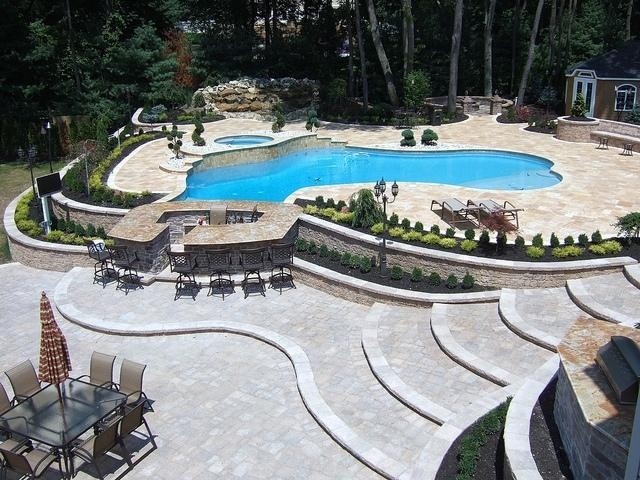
622;142;635;157
598;137;609;150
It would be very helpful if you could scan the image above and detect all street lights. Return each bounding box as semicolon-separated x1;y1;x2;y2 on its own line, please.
17;146;42;208
373;177;399;278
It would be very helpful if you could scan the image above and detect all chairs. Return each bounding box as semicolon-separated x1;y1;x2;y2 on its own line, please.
0;350;158;480
82;238;144;296
165;240;296;301
431;198;524;231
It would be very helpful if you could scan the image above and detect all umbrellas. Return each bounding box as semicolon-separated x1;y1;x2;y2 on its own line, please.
37;290;74;436
